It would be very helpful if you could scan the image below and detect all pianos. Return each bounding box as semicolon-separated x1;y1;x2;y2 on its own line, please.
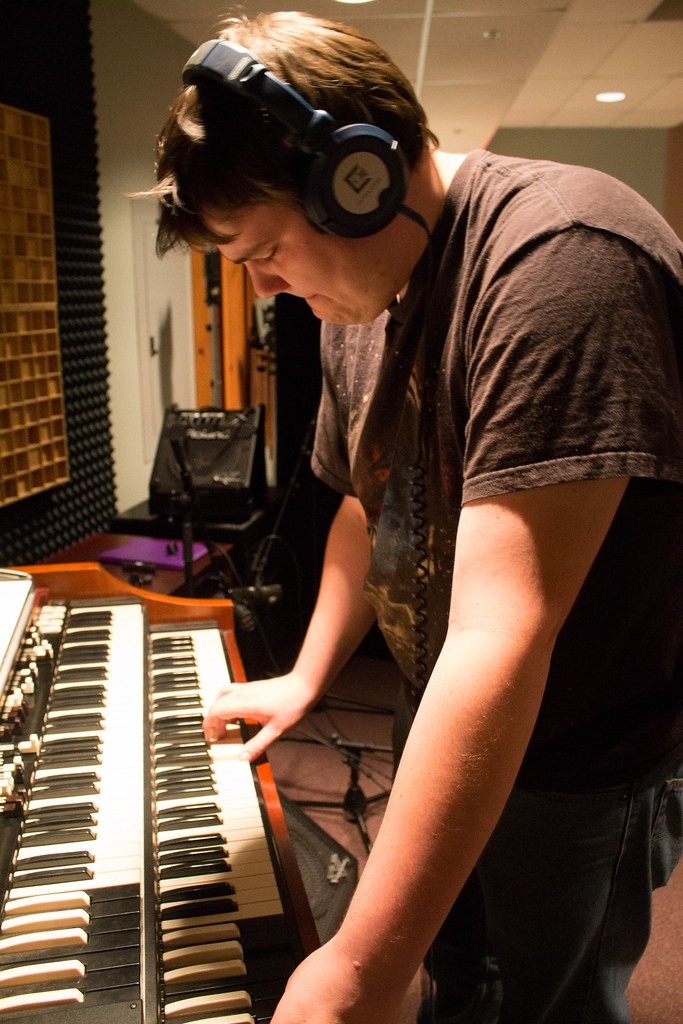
0;546;322;1024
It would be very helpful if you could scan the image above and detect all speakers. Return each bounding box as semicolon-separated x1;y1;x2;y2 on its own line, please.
149;404;267;523
276;793;358;947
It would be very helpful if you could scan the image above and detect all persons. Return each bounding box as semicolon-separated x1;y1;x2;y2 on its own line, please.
153;11;683;1024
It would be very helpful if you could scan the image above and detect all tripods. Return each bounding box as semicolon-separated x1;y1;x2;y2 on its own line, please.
281;735;394;856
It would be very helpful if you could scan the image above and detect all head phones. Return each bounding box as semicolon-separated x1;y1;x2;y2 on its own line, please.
180;38;408;238
